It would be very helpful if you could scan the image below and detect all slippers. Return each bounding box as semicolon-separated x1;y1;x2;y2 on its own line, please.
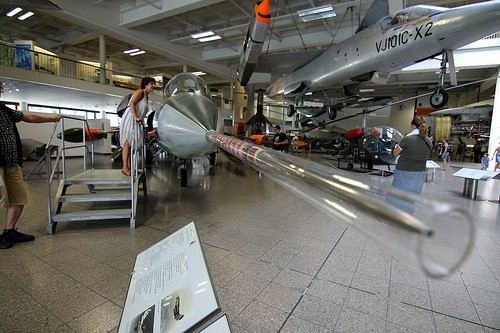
121;170;131;176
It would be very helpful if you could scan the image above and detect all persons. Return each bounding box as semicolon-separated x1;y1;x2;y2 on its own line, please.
482;153;492;170
440;138;450;170
0;81;63;249
120;77;156;176
272;125;288;151
492;143;500;172
392;116;432;194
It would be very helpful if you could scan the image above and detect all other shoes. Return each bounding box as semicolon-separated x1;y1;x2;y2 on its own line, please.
442;169;445;171
1;227;35;242
0;235;12;248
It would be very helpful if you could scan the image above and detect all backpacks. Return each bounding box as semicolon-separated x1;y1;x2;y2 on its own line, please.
117;89;144;117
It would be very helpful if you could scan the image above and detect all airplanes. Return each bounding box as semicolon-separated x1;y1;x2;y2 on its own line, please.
27;0;500;173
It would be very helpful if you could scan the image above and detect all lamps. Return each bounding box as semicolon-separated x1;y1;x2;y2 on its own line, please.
296;5;337;22
189;29;223;43
123;48;145;56
